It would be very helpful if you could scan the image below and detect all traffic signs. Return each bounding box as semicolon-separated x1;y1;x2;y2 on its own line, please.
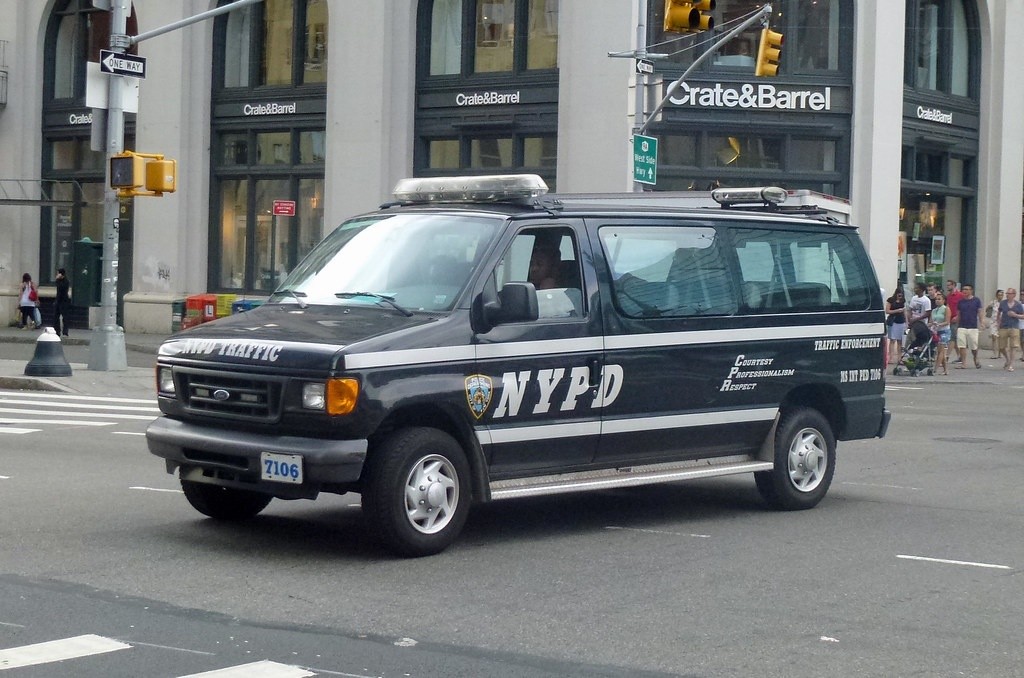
273;199;296;217
635;57;655;76
631;133;657;185
99;48;147;80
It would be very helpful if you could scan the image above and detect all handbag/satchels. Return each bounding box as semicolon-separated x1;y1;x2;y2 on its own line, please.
886;314;896;326
34;307;42;326
29;282;37;301
986;300;995;317
15;309;23;323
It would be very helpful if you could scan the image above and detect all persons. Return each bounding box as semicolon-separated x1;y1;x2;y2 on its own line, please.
53;268;71;337
1018;288;1024;362
928;294;952;376
529;239;574;290
886;287;908;364
996;288;1024;371
955;284;984;370
946;280;965;363
17;272;40;331
907;282;941;326
985;290;1007;359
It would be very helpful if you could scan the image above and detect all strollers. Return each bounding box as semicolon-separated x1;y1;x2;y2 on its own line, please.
892;320;938;377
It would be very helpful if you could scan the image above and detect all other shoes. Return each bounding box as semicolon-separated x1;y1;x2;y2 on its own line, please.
952;359;962;363
1007;365;1014;371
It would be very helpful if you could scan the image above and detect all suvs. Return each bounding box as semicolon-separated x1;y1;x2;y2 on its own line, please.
144;173;892;557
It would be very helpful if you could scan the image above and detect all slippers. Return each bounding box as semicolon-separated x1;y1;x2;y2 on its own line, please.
976;363;981;369
956;366;966;369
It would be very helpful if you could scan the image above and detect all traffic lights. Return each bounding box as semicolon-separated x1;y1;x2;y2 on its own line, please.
753;26;784;79
663;0;702;34
686;0;716;34
109;153;145;190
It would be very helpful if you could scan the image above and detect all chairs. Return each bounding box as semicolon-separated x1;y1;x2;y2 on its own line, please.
684;248;837;318
